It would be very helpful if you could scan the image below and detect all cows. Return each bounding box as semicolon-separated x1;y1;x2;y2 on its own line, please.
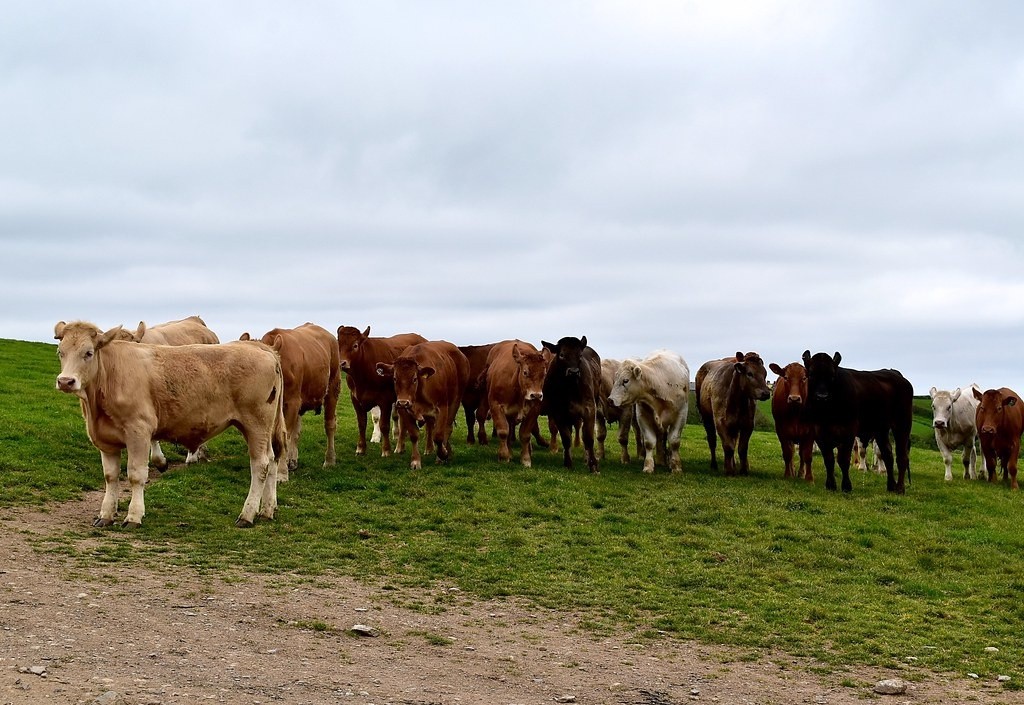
929;382;988;481
692;351;771;477
972;386;1024;492
48;312;691;532
768;350;914;495
